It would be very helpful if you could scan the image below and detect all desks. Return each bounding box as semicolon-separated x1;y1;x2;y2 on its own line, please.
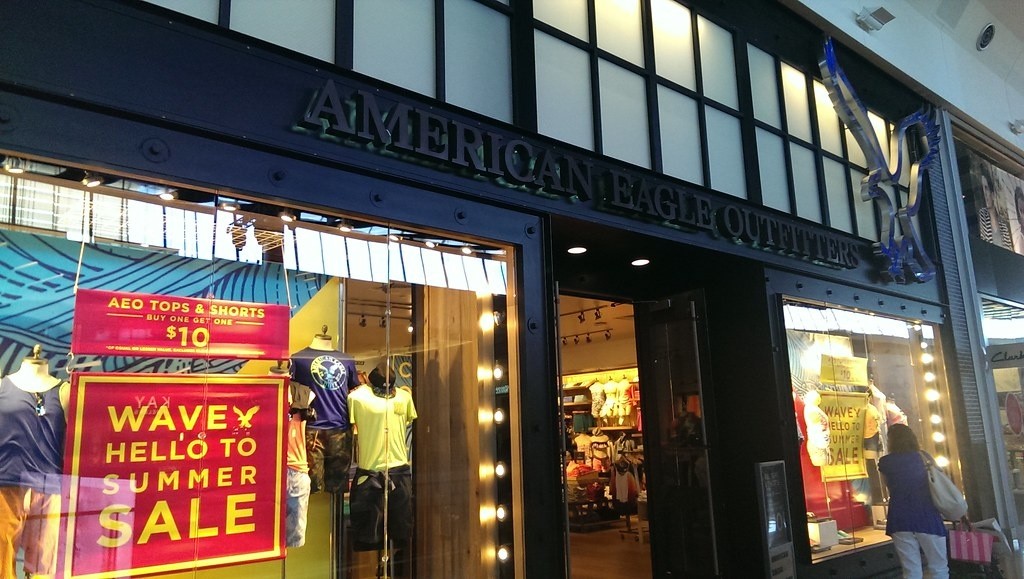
1006;445;1024;492
637;498;650;545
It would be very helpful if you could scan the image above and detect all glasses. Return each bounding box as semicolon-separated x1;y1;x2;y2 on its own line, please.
34;392;45;417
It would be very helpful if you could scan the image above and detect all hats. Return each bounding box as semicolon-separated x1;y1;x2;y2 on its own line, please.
368;363;396;388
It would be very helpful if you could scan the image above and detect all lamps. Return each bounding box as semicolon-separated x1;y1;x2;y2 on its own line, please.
359;316;387;329
0;156;475;257
559;305;614;344
857;5;897;33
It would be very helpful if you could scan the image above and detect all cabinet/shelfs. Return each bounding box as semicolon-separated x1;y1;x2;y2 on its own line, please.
588;426;637;441
558;379;592;451
629;381;642;438
559;475;628;532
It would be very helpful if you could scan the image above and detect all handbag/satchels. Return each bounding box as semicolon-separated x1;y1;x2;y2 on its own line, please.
917;450;968;521
948;515;994;563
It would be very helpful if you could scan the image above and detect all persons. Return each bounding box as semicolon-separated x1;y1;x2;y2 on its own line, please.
877;424;950;579
565;372;635;418
288;335;361;493
344;365;418;549
977;161;1024;254
1;355;71;579
263;368;316;548
793;374;909;506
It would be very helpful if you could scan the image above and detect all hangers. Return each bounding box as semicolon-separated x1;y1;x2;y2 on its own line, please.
610;451;645;467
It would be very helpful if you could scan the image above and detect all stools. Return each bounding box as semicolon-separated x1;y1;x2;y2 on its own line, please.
1010;469;1020;490
